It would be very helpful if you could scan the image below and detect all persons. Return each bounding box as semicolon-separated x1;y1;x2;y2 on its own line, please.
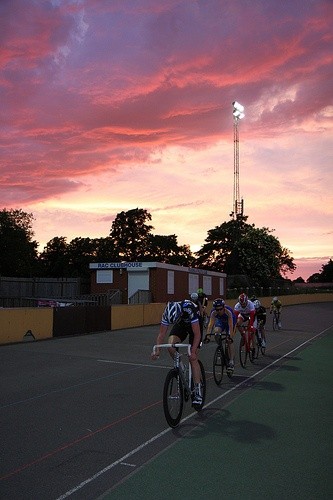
151;300;204;406
270;297;283;328
190;288;266;372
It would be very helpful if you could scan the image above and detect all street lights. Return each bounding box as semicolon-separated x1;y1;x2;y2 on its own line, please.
232;99;246;220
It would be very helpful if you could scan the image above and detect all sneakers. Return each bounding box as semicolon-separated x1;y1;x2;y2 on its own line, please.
261;340;266;347
228;362;235;371
180;361;186;372
192;394;203;406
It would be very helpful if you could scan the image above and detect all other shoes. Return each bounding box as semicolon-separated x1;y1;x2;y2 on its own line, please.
278;321;282;328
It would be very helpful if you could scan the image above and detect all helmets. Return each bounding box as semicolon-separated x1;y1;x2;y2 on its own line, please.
197;288;203;294
164;301;183;325
212;297;226;307
238;292;248;305
272;295;278;302
190;292;198;301
253;300;261;308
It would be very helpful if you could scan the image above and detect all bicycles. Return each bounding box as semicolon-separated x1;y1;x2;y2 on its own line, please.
239;324;259;366
205;331;235;385
202;313;209;329
255;329;267;357
268;308;280;331
150;341;208;429
